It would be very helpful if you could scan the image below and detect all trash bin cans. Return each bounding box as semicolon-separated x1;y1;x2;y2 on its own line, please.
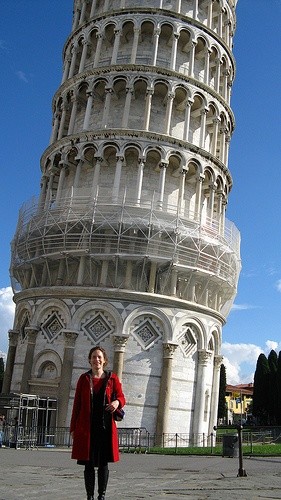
221;433;240;459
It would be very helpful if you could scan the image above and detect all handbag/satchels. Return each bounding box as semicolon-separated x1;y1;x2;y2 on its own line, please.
113;407;124;421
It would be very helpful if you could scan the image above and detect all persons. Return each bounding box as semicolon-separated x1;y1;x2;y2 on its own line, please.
0;414;23;449
68;346;126;500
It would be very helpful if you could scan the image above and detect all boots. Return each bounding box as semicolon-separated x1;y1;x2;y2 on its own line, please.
83;470;95;500
97;470;109;500
